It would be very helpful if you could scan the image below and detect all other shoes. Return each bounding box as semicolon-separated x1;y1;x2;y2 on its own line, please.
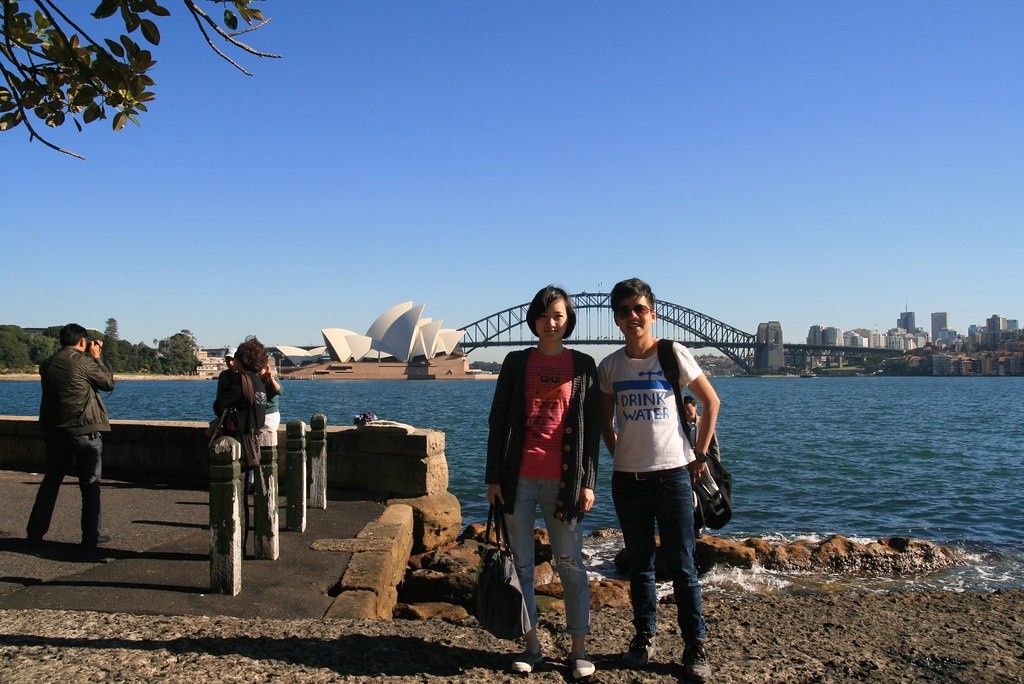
81;534;110;544
25;536;42;547
571;653;596;679
512;650;543;673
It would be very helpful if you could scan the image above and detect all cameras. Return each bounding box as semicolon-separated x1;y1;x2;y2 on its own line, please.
85;342;91;352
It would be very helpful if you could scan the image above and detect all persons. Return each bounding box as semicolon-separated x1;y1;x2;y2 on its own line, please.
213;335;269;560
683;395;720;539
483;284;601;678
25;322;114;546
596;278;720;684
222;347;238;368
258;364;284;433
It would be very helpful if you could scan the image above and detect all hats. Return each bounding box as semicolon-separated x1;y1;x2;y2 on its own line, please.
225;348;236;358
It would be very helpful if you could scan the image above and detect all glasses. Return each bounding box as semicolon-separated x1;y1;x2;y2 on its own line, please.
615;305;652;319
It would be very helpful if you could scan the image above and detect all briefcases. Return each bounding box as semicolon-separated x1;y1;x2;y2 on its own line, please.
233;435;260;470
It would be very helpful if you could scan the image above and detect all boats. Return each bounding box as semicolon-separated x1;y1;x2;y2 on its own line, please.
799;370;816;377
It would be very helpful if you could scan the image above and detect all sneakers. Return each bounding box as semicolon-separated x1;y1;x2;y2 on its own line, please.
681;637;711;683
623;632;657;665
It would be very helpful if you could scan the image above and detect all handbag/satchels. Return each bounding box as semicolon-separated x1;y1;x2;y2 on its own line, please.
471;494;532;641
689;447;733;531
204;415;221;439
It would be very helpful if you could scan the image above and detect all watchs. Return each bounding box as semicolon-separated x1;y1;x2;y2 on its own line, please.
693;451;709;463
